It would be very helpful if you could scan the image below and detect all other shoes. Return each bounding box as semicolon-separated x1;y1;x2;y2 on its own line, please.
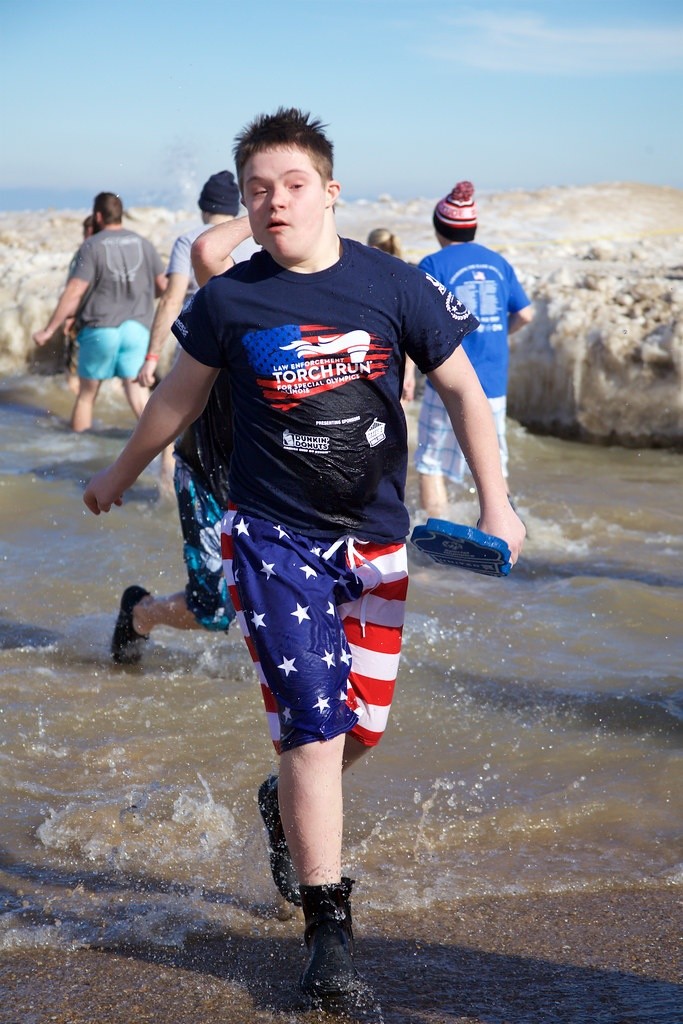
112;582;152;665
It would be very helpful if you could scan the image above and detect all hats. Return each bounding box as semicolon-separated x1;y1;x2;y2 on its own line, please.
434;182;478;243
199;171;240;217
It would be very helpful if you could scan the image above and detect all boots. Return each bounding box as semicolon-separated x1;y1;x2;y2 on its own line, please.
254;777;360;1006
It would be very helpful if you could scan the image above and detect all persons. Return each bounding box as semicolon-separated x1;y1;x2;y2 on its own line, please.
107;214;263;663
81;108;529;1016
367;227;414;402
31;191;169;432
410;181;534;520
63;214;96;396
127;169;263;494
